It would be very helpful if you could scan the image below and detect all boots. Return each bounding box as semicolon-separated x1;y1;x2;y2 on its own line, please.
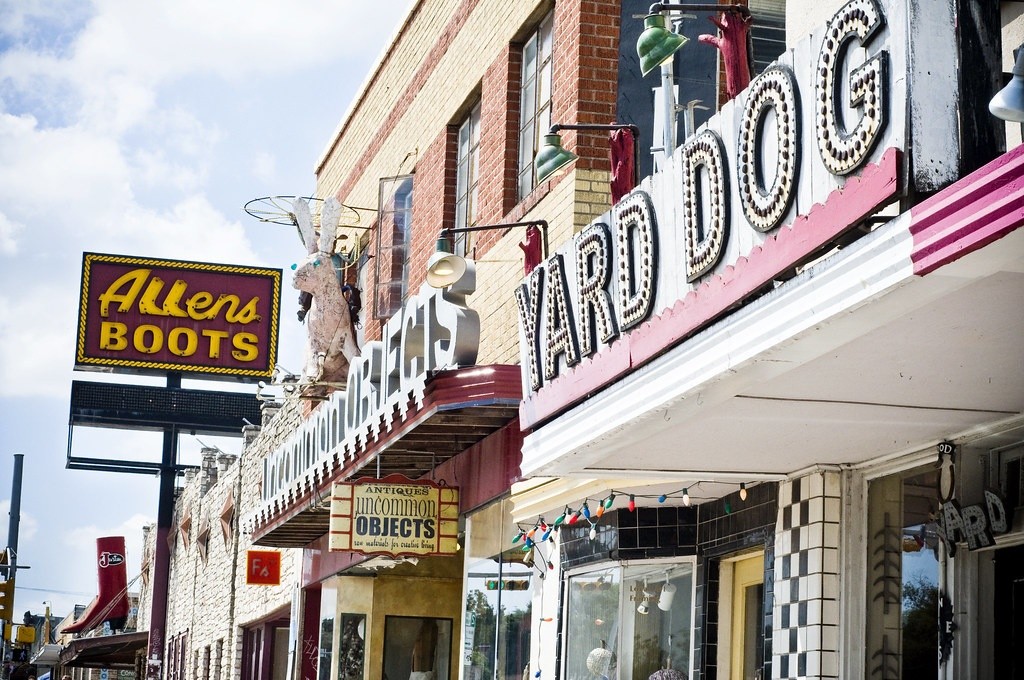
61;536;129;633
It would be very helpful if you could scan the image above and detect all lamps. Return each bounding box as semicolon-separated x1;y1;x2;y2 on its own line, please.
423;220;550;290
647;607;690;680
989;43;1024;124
637;575;656;614
586;639;617;678
537;122;643;191
357;619;365;641
256;351;347;401
635;3;758;80
658;570;677;612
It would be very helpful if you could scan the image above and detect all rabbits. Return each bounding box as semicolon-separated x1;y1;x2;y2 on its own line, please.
290;195;362;377
